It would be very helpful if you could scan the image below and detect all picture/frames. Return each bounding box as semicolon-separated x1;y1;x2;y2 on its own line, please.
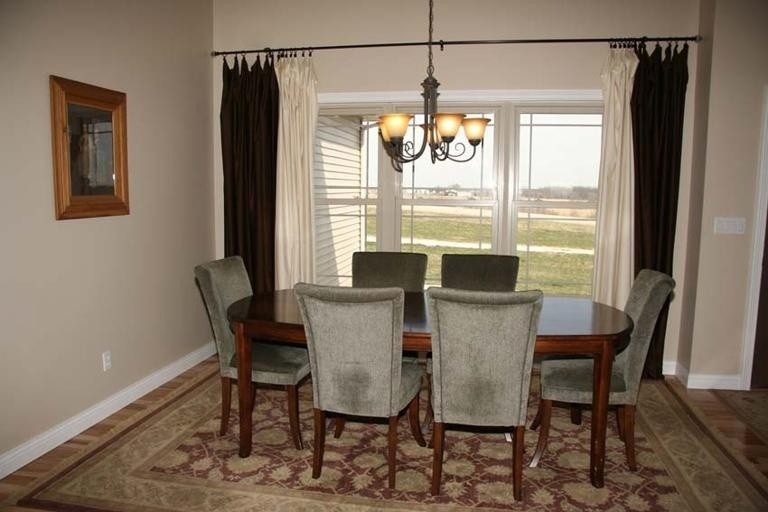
49;74;130;224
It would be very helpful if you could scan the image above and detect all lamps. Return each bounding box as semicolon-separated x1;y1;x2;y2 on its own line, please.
377;1;493;172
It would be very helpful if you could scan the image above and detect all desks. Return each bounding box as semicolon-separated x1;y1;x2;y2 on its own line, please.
227;287;636;490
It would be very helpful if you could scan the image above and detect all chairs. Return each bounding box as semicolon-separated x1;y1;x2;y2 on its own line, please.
294;282;425;491
440;254;523;291
327;254;432;447
194;255;341;453
423;287;543;501
528;268;676;472
